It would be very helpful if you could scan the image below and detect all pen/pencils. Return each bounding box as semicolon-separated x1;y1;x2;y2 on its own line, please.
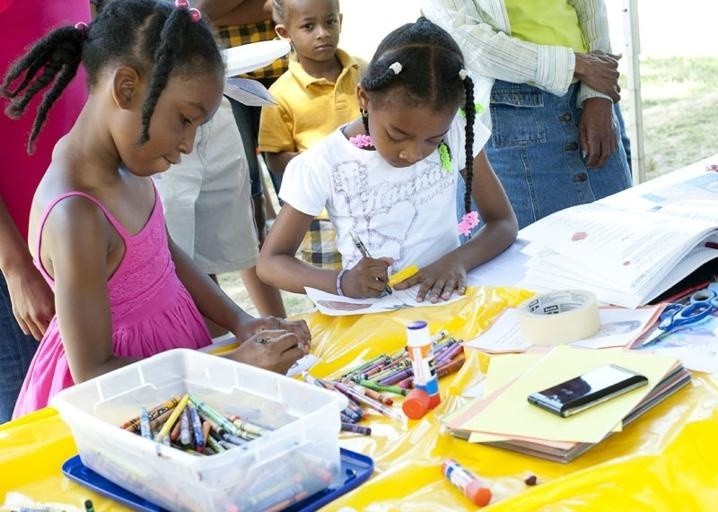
300;330;466;435
257;336;299;366
85;394;339;512
353;235;393;295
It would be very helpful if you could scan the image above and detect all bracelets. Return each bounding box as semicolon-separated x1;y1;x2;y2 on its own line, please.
334;266;349;297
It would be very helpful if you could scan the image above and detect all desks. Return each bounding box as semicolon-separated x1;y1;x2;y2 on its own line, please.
0;149;718;512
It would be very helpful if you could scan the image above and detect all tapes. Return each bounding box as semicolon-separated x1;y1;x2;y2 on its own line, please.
517;288;600;342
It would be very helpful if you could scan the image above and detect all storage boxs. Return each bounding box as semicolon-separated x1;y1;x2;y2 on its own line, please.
46;347;347;512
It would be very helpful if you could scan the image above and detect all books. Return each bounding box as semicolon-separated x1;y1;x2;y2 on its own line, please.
521;170;717;309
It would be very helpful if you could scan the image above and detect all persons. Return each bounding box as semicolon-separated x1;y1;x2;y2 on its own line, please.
2;0;310;425
259;1;370;269
145;97;288;339
253;17;521;302
0;0;94;432
418;2;634;235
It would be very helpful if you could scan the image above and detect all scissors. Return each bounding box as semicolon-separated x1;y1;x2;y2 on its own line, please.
690;287;718;308
641;301;713;346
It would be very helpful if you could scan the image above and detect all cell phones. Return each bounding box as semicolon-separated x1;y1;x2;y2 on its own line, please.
526;363;648;416
